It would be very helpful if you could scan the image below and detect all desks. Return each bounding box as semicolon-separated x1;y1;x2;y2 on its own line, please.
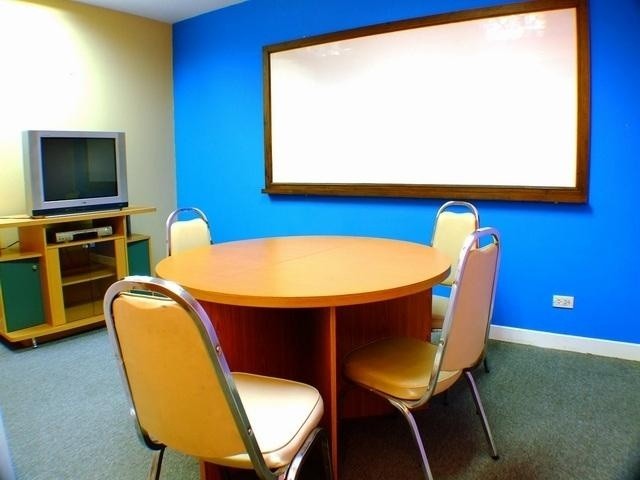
155;235;451;480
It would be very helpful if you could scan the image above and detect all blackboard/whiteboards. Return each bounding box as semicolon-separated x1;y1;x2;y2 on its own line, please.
263;0;590;203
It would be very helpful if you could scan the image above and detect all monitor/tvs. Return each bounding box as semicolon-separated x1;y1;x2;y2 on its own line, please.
22;130;128;219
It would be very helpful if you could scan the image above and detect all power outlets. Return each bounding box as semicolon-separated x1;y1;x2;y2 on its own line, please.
551;294;574;310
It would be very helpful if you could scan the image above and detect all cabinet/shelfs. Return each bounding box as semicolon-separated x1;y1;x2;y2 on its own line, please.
0;206;157;350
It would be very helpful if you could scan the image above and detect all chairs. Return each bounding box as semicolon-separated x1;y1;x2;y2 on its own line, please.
420;199;480;333
338;227;501;480
165;206;214;257
103;274;333;480
